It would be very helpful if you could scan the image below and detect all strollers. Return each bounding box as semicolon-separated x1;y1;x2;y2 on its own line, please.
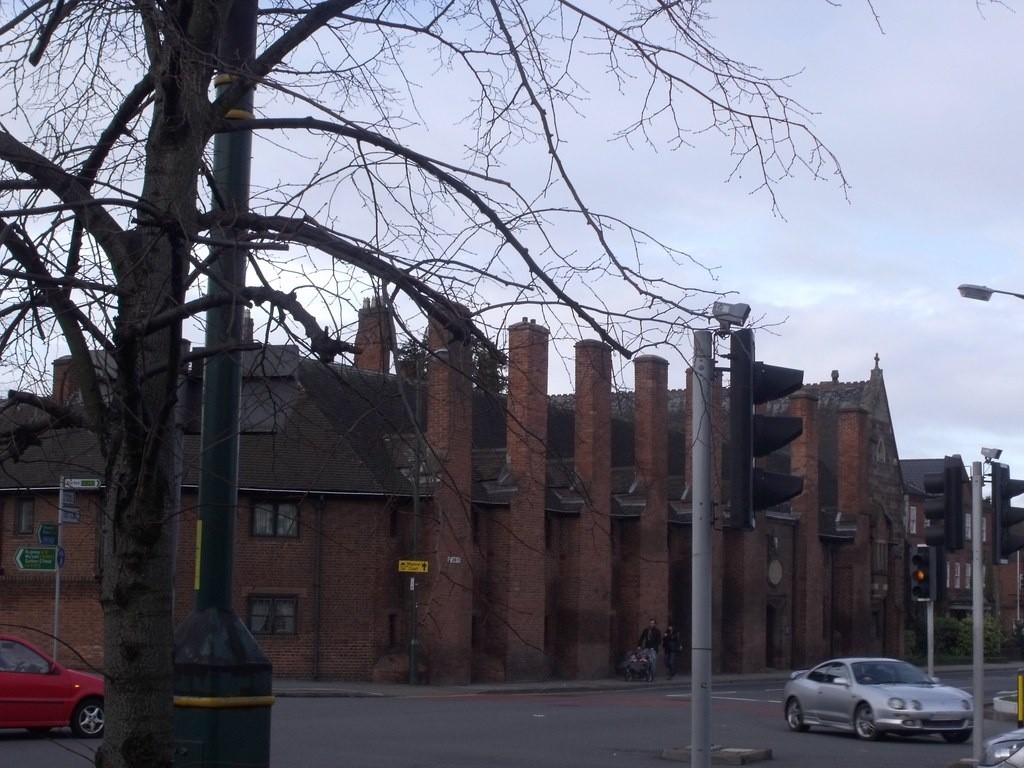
625;646;657;681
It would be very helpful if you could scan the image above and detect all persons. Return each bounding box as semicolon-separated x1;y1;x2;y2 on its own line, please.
629;654;648;662
636;618;662;653
662;624;682;680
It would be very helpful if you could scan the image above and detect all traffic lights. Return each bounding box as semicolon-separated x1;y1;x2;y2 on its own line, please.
991;463;1024;567
923;456;966;549
725;327;803;532
911;547;934;602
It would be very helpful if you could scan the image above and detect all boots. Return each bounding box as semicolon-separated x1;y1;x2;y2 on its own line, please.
667;668;673;680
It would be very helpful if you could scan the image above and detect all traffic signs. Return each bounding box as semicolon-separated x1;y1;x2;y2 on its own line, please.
14;476;101;572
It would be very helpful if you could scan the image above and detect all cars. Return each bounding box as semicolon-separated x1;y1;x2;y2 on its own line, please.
783;655;975;742
0;634;104;739
977;728;1024;768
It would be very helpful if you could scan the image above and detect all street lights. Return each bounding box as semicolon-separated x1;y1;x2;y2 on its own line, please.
408;348;450;683
